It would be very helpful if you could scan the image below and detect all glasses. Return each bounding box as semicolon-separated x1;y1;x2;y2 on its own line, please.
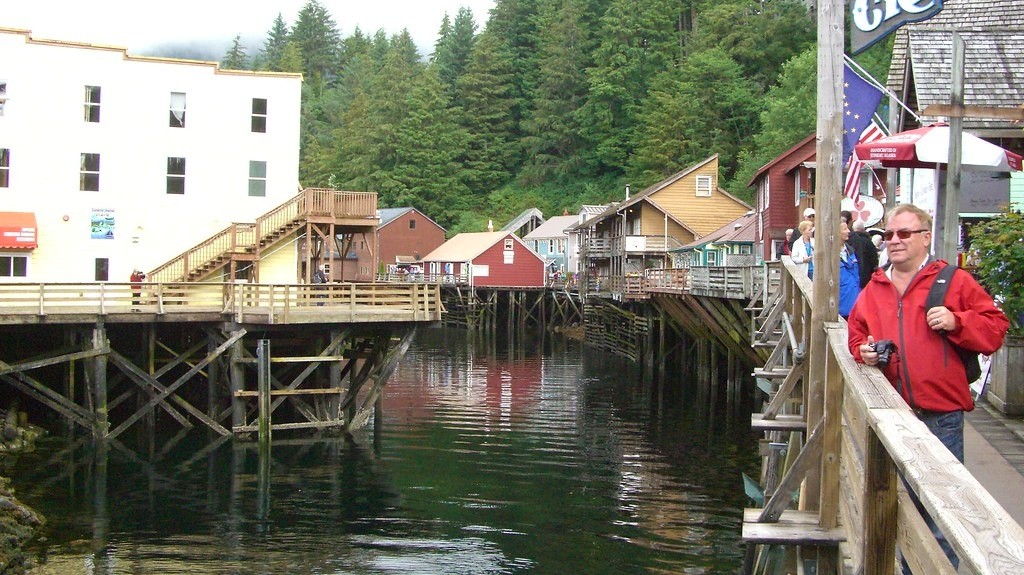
807;216;815;218
884;228;930;241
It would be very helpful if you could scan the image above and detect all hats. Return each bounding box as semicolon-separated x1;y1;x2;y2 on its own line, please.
804;208;816;216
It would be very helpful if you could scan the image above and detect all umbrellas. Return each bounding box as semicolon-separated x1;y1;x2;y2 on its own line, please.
853;120;1022;172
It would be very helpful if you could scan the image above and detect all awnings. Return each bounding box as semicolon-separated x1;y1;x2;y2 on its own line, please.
1;211;38;250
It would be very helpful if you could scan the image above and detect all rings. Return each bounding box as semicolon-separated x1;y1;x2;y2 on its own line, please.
936;318;940;324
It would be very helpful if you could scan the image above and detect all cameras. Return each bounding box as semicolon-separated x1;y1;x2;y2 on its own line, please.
868;339;897;367
138;272;145;279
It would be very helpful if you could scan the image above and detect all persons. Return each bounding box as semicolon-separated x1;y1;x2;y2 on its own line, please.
386;263;452;284
840;211;866;292
777;229;794;260
852;220;878;288
130;270;142;313
791;220;816;283
313;264;329;305
545;268;579;288
840;216;862;323
848;203;1011;574
788;208;816;253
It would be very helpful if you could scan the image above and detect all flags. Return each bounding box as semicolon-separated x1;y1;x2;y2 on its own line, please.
843;57;885;174
840;117;890;207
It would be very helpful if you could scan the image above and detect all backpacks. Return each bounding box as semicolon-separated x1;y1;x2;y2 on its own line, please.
924;264;981;384
